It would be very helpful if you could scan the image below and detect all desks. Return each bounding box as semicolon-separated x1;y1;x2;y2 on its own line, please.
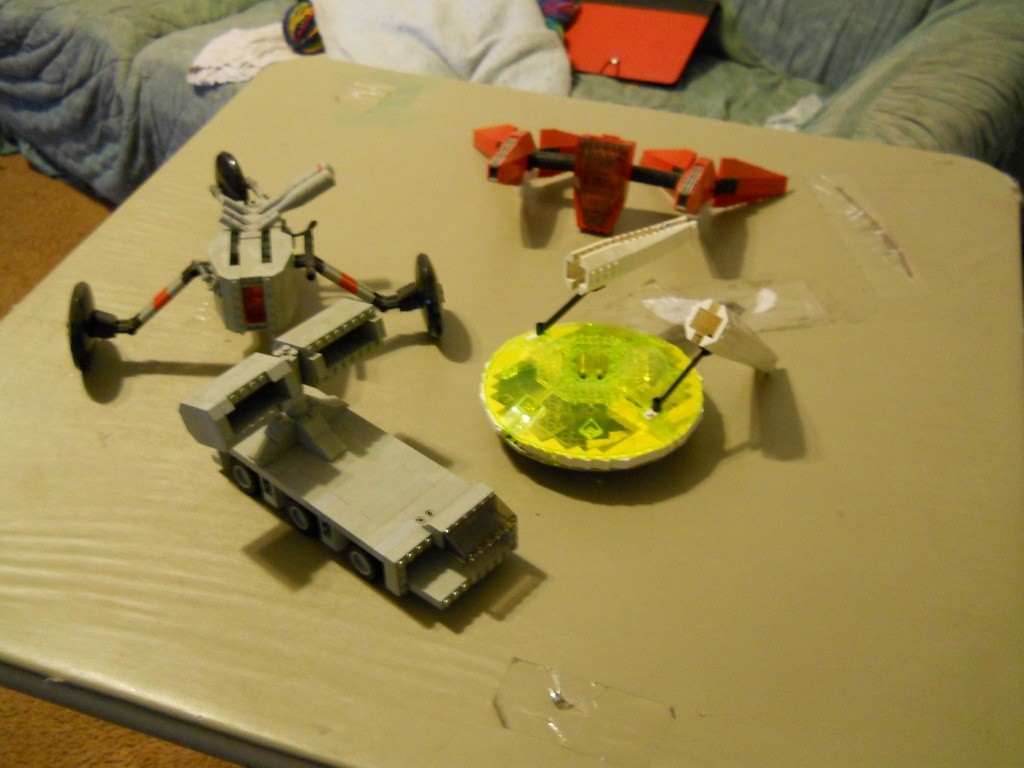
0;52;1023;766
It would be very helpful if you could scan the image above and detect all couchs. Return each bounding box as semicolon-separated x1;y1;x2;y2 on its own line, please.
0;1;1023;264
